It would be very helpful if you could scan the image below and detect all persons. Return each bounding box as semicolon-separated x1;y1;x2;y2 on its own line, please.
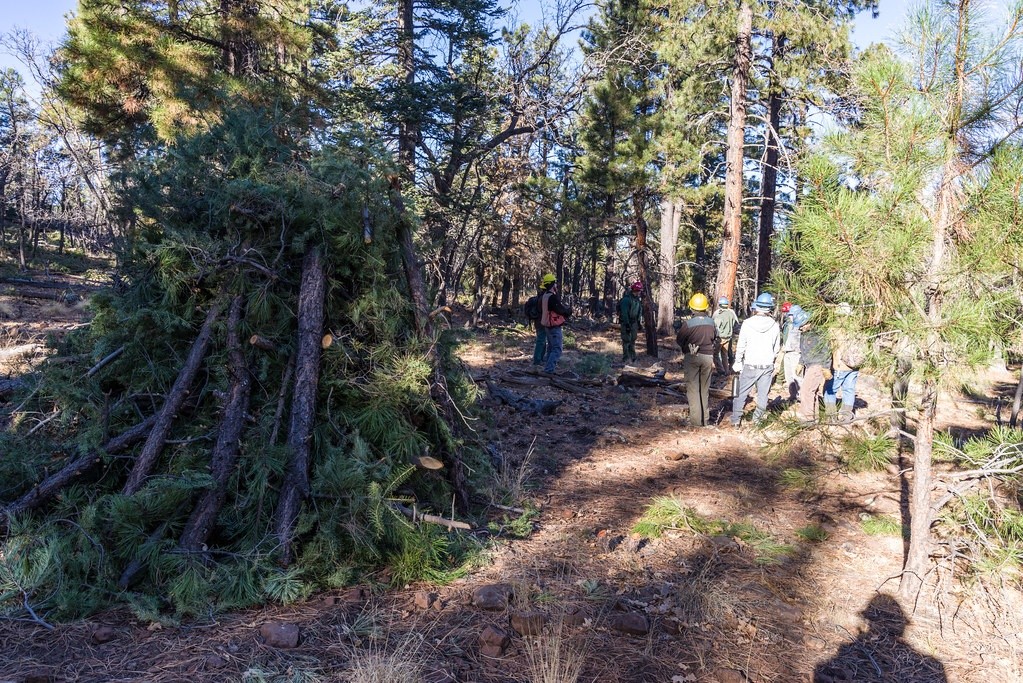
730;292;780;429
619;282;644;362
772;302;803;403
676;293;717;426
793;310;833;427
534;284;547;365
823;302;860;420
541;274;572;375
712;295;739;376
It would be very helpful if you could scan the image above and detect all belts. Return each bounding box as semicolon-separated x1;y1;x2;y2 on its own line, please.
786;351;794;353
720;337;730;339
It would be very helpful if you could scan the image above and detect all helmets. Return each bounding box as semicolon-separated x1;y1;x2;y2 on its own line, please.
689;293;708;310
780;303;793;311
631;281;643;291
794;311;811;327
718;297;728;305
543;274;556;282
755;293;775;307
787;305;802;315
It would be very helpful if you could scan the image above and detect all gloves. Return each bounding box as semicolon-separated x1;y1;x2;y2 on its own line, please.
823;369;833;380
732;361;742;372
688;343;699;355
795;364;804;377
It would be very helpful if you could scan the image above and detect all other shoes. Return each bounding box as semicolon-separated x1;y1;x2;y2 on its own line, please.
683;420;696;427
731;424;739;429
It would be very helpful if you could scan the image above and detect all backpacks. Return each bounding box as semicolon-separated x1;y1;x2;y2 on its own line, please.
616;295;640;317
524;292;541;319
836;326;868;369
